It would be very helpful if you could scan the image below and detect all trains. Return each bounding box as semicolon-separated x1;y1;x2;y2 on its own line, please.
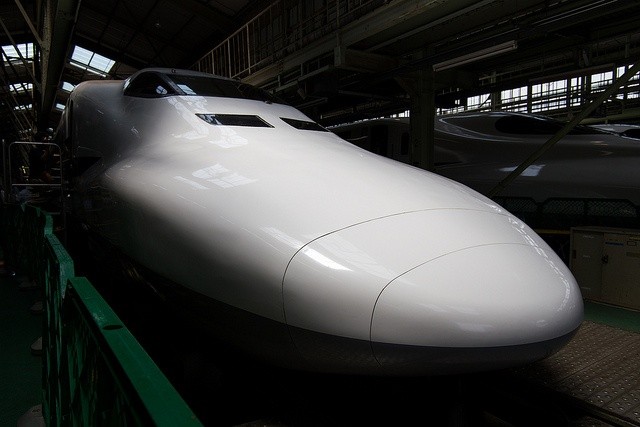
42;67;585;375
326;111;640;217
